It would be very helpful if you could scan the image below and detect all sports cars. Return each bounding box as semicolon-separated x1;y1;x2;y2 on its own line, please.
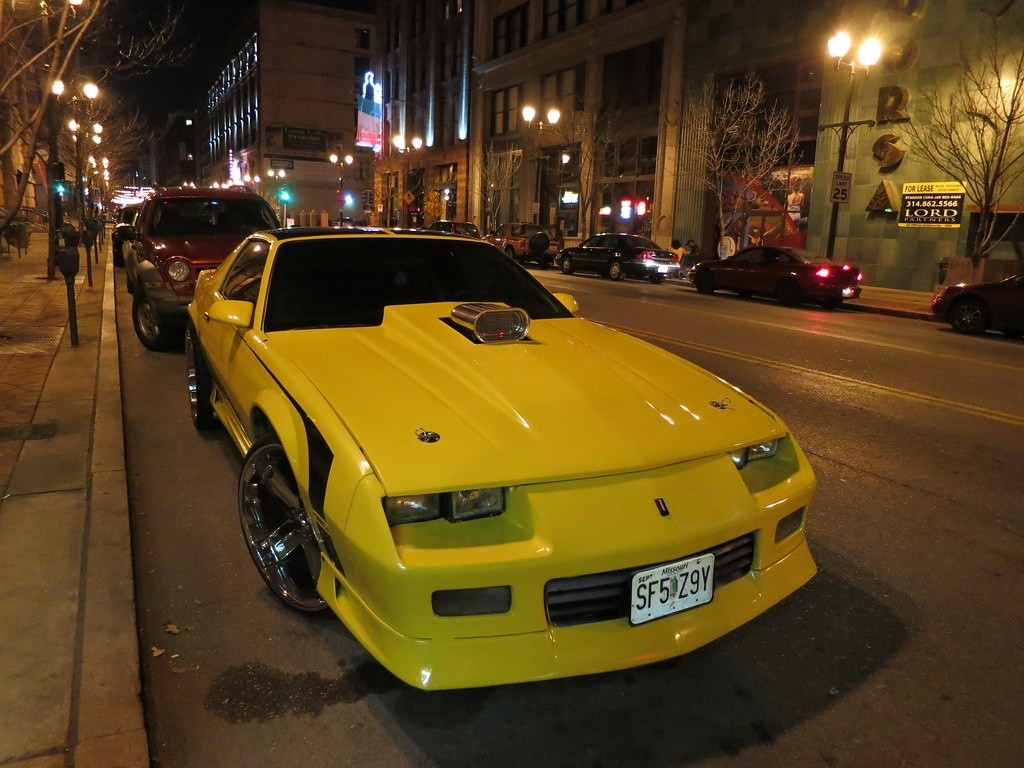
183;226;821;693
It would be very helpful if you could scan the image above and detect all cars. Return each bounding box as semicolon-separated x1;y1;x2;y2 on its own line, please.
687;244;864;311
111;201;144;268
554;232;681;285
929;272;1024;342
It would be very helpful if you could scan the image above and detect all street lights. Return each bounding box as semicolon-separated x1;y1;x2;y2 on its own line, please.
267;168;286;223
328;153;354;226
392;135;423;229
815;23;884;261
519;105;561;224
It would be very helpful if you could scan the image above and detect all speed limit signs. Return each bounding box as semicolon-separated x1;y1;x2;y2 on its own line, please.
830;170;853;205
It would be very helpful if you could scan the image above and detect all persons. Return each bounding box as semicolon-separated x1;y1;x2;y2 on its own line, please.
666;239;690;265
686;239;700;257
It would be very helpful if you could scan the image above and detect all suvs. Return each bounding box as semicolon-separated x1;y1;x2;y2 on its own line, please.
116;184;284;352
427;220;481;240
479;222;564;267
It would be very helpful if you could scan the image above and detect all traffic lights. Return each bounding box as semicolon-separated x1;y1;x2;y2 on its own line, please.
52;162;68;198
369;191;374;205
361;191;368;205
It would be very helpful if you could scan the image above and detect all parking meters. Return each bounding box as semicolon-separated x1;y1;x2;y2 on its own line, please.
86;218;101;265
82;229;95;287
55;245;80;347
